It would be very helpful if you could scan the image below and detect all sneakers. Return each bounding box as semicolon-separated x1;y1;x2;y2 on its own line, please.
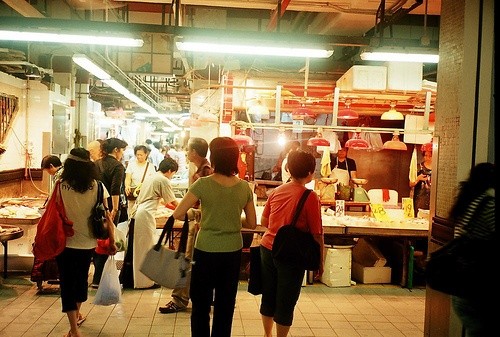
158;300;188;313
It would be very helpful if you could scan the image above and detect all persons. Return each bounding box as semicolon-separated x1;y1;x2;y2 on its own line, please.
89;131;190;228
258;142;302;189
90;138;127;288
158;137;213;314
173;135;257;337
51;147;118;337
120;158;179;289
328;140;357;193
260;151;324;337
41;154;64;190
451;161;500;337
408;150;432;258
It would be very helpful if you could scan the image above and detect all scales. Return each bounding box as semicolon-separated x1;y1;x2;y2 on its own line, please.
319;178;371;202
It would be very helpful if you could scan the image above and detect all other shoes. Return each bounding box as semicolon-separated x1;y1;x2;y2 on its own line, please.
140;283;161;290
63;330;81;337
76;313;85;325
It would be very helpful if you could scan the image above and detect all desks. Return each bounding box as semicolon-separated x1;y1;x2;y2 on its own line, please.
0;197;51;258
0;223;25;279
155;196;430;288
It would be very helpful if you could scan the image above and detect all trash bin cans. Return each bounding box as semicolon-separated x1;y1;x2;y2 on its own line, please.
320;244;356;287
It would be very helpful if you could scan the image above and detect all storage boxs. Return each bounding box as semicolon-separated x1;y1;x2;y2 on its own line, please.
388;63;424;92
335;66;388;92
322;248;351;287
368;188;399;206
352;266;393;284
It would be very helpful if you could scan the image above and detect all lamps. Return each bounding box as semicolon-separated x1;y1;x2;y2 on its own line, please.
0;28;146;49
247;100;270;116
337;100;359;120
359;46;441;64
72;54;189;132
382;100;405;121
277;127;288;147
306;129;331;147
344;132;371;150
176;34;335;59
380;131;409;151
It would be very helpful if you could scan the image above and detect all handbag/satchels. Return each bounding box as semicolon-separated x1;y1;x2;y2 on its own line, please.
127;187;141;199
248;244;262;295
90;180;111;240
272;225;321;271
426;233;500;302
139;210;190;290
29;241;60;281
56;198;75;238
91;254;122;306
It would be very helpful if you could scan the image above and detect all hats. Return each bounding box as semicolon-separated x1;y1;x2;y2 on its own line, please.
68;149;90;163
